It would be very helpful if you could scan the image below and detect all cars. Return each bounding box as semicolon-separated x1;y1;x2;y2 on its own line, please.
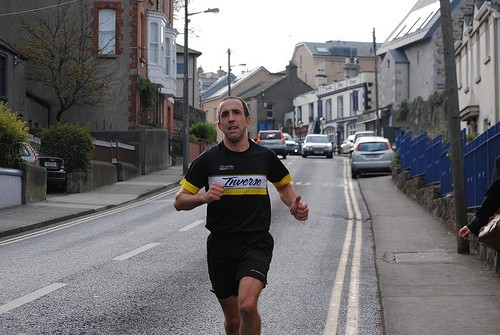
350;130;396;179
18;141;66;195
267;132;299;155
340;134;355;154
301;134;333;158
256;129;288;159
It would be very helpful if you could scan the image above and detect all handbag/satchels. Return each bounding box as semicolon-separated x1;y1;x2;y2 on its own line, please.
478;214;500;254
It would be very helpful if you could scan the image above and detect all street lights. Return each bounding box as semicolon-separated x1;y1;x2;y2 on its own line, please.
180;0;220;175
228;47;247;97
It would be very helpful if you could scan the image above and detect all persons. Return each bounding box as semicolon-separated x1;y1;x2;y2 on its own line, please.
459;178;500;241
173;97;310;335
